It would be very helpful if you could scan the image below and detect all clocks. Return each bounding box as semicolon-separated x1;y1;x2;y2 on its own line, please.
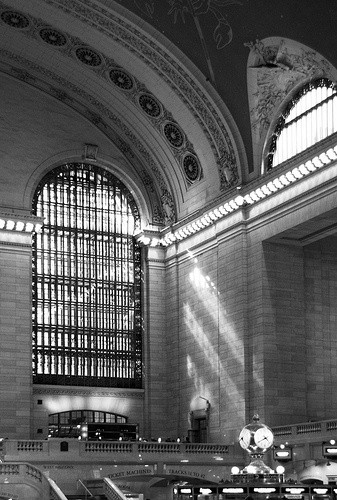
237;413;274;475
